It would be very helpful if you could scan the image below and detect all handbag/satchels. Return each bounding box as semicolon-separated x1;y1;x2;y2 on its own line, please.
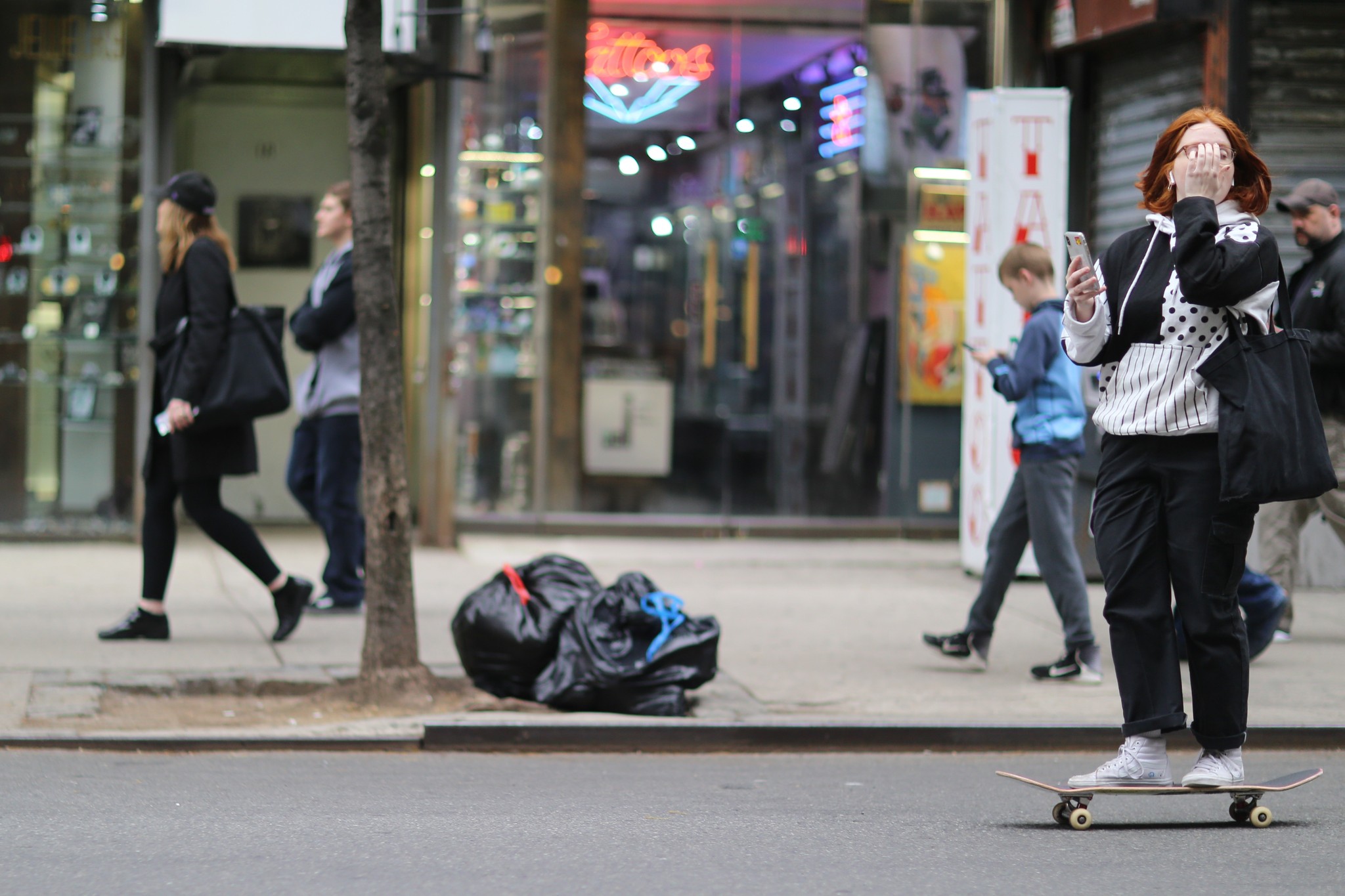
536;570;721;717
1196;248;1337;504
452;554;604;702
147;305;290;427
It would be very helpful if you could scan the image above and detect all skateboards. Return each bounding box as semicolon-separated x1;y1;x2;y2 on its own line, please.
995;768;1324;830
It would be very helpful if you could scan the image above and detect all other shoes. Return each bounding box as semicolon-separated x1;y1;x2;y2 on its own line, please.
1245;598;1288;658
303;594;362;614
272;577;313;641
98;606;171;640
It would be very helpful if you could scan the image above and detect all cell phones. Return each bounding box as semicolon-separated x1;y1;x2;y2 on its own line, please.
1064;231;1100;292
154;405;200;436
961;340;978;351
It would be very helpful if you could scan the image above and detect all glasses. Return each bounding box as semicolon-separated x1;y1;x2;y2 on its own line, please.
1172;144;1238;165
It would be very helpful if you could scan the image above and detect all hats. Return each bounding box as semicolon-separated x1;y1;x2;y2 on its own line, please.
153;171;218;215
1276;177;1340;213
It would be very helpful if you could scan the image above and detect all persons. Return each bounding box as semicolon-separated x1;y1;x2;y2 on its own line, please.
1059;105;1285;790
285;177;366;615
921;240;1102;685
97;168;314;642
1256;177;1345;643
470;329;505;510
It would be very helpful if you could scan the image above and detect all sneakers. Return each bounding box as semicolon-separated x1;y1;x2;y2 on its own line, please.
1180;742;1245;787
1031;645;1102;685
923;627;992;669
1067;734;1173;789
